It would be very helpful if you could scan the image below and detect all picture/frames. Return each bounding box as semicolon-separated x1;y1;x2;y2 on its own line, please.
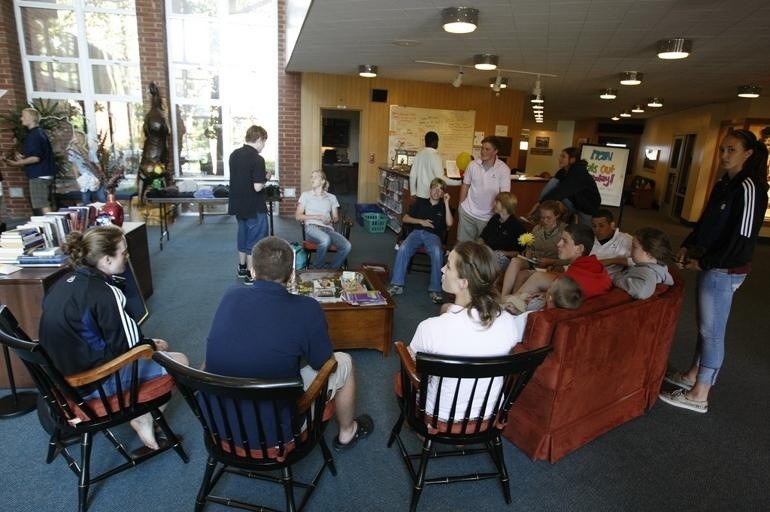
536;136;551;148
112;253;150;330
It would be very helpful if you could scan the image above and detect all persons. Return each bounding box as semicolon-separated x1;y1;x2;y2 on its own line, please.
34;224;189;452
456;135;512;245
387;177;454;304
394;239;548;448
657;128;769;414
294;169;352;268
408;131;464;206
526;146;602;229
438;190;694;315
194;235;375;461
227;125;274;286
4;108;59;215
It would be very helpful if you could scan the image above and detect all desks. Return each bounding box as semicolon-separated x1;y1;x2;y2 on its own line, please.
289;267;396;357
49;187;139;219
0;218;155;390
147;188;282;251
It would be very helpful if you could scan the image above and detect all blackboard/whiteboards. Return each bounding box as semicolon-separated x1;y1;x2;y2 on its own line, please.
577;143;632;208
387;104;476;170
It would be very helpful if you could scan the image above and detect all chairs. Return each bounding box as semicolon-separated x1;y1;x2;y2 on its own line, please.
195;182;235;227
300;201;354;271
0;301;188;511
384;340;554;512
150;348;339;511
399;207;450;274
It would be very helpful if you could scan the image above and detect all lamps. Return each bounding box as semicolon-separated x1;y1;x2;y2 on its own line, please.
143;159;168;190
620;95;666;120
735;84;761;99
358;65;378;78
473;53;498;71
489;77;508;89
530;95;544;123
441;6;478;34
448;66;542;95
618;71;643;86
599;88;617;100
655;38;691;60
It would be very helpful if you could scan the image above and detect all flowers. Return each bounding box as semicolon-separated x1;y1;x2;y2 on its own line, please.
62;130;134;192
516;232;536;252
74;171;100;194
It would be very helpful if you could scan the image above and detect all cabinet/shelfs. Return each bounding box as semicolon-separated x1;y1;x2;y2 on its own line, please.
376;165;411;237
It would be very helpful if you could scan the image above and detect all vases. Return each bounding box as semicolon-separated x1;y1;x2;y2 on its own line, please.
103;192;125;229
79;190;92;204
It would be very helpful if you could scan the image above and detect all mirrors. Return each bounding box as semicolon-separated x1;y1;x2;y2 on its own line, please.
643;148;660;174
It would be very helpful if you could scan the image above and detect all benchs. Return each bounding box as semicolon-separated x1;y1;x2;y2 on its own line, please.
622;172;657;210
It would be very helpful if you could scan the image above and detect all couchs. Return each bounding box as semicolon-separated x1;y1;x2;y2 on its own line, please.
502;268;689;462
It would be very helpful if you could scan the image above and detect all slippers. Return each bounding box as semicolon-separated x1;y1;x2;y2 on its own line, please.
332;414;374;453
130;433;184;459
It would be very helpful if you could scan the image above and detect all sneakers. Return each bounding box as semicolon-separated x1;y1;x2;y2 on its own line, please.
429;291;444;304
237;264;247;277
385;285;404;297
243;271;257;285
658;388;708;414
665;367;696;391
